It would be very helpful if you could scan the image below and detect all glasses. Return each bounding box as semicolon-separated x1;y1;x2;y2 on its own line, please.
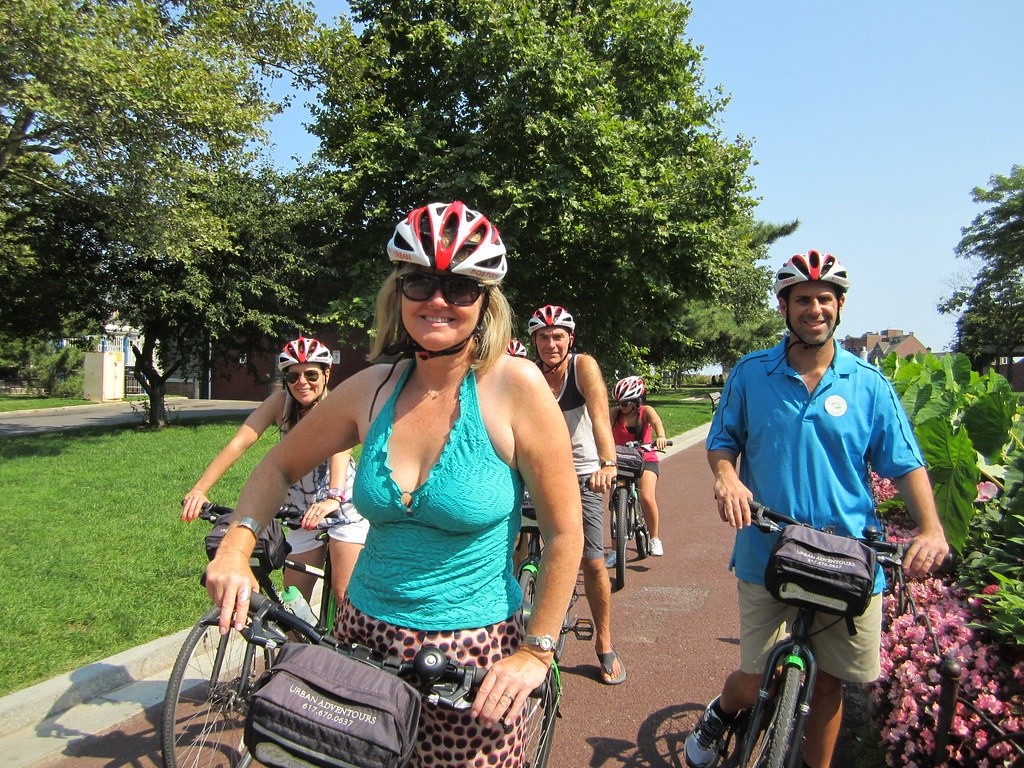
284;370;323;384
398;271;485;306
617;402;634;407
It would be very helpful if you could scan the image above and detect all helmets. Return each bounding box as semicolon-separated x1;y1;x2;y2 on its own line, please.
527;304;576;336
773;250;849;297
386;200;508;282
277;337;333;372
611;376;645;403
504;339;527;357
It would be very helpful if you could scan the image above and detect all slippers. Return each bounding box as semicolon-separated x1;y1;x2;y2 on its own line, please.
596;643;626;684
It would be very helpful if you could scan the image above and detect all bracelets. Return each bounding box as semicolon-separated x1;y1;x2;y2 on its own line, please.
326;487;345;502
328;497;341;504
194;487;205;494
237;516;262;543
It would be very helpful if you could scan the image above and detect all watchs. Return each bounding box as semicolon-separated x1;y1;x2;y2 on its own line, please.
523;635;558;652
601;460;618;468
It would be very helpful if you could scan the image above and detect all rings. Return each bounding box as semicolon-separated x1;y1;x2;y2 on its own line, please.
504;691;515;701
313;513;319;517
927;554;934;559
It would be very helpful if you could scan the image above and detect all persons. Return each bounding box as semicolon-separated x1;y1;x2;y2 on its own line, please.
207;200;584;768
506;339;527;358
514;304;627;685
182;336;370;641
683;249;949;768
604;376;668;568
712;375;718;384
718;374;724;385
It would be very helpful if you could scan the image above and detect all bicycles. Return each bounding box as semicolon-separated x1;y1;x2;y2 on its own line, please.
713;493;955;768
160;496;337;768
201;567;549;768
511;471;619;664
609;438;675;592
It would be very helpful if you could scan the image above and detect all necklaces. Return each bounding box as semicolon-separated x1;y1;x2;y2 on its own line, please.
414;374;463;397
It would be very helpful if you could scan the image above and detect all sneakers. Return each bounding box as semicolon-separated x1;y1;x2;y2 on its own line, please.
605;550;616;568
683;693;734;768
649;538;663;556
